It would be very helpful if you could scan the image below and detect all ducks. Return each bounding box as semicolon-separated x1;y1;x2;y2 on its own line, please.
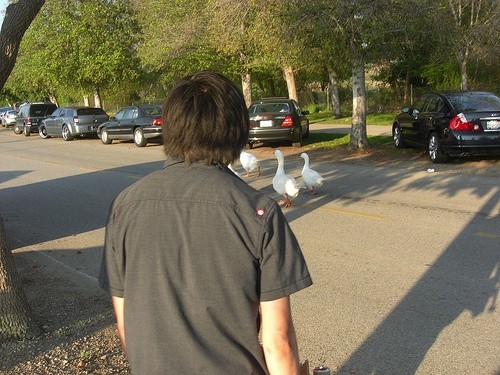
240;151;260;177
272;150;299;207
298;152;326;194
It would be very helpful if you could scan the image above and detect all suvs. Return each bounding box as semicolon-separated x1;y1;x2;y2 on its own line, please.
12;102;60;137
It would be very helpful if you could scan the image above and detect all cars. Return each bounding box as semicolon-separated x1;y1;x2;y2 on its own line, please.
392;90;500;163
96;103;165;148
2;111;18;129
246;97;309;149
0;106;14;120
38;106;112;141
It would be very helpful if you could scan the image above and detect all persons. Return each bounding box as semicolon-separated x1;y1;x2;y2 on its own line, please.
97;69;317;375
